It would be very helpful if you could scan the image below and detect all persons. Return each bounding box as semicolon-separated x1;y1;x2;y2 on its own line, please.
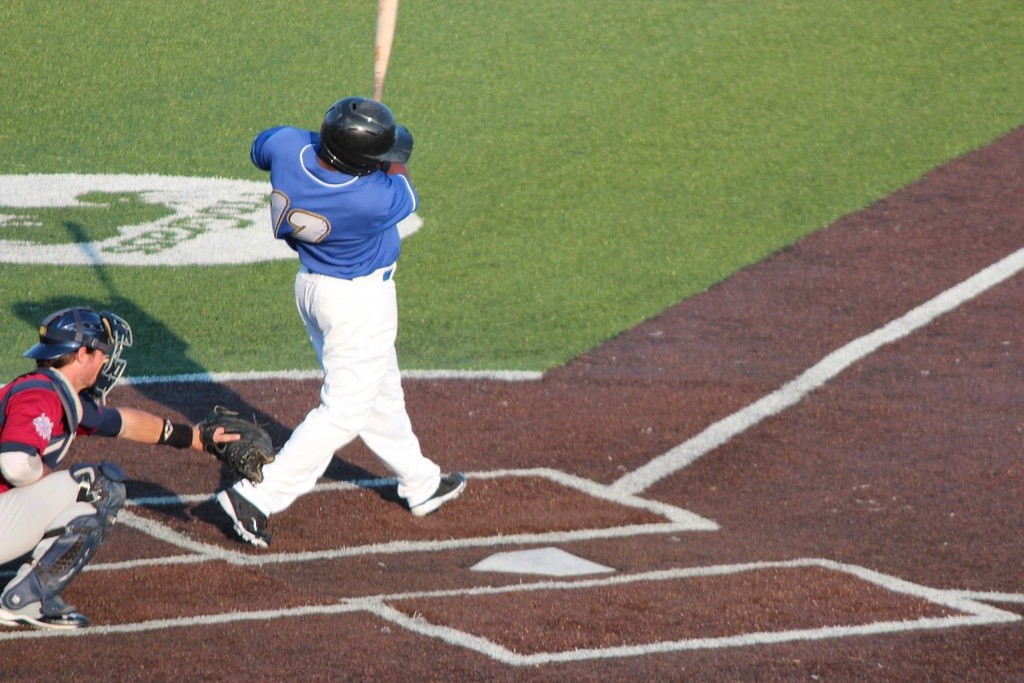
1;306;243;633
216;97;469;549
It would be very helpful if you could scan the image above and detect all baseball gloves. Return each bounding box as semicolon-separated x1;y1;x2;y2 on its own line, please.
201;405;275;484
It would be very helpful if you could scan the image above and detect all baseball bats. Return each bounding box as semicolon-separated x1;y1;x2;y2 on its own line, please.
371;0;398;101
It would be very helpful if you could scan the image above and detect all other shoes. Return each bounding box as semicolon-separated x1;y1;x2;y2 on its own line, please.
0;562;89;629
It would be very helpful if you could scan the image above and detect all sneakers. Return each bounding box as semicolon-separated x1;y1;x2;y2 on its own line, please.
410;472;466;516
216;487;269;550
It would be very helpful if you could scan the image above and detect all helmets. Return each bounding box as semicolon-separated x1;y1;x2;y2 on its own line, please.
23;306;107;361
316;98;414;176
